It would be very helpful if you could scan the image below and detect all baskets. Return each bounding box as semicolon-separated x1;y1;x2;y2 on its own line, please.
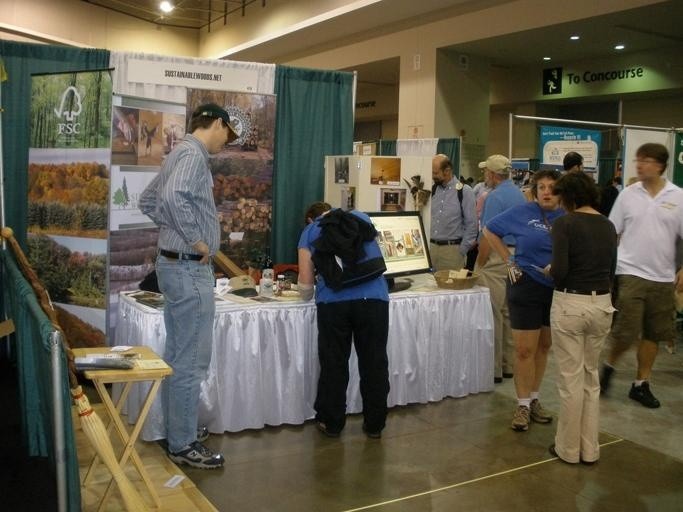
433;269;480;289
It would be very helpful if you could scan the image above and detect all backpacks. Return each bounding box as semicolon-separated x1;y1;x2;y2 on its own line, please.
312;209;387;290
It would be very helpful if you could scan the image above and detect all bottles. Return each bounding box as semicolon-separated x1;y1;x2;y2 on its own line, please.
277;275;285;290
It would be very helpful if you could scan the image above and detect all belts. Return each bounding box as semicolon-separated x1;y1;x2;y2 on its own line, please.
158;250;203;260
508;244;514;247
552;287;611;295
430;239;461;245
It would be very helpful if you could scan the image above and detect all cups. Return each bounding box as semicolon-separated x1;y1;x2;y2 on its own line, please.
259;279;274;297
261;268;274;278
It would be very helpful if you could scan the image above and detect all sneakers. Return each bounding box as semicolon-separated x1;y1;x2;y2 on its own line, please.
530;399;553;423
600;362;614;393
314;419;339;437
628;381;660;408
512;405;531;431
166;442;224;468
197;425;210;442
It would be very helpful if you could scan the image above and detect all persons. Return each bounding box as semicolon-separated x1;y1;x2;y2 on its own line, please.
513;168;525;184
614;176;622;192
116;113;136;147
298;201;390;439
396;242;403;252
465;183;494;277
428;153;477;272
598;184;617;218
540;171;616;464
473;179;490;197
143;120;160;155
137;104;239;468
482;172;564;430
598;143;683;409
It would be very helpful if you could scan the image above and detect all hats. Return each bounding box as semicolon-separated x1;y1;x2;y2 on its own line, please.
228;276;257;296
479;154;512;175
190;104;240;143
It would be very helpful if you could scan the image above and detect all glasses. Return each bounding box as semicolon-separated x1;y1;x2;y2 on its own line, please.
632;159;657;165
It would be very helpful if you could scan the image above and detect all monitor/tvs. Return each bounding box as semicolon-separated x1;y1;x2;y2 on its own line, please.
364;212;433;293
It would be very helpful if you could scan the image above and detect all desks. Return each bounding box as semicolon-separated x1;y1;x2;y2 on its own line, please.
72;344;174;512
112;284;495;445
